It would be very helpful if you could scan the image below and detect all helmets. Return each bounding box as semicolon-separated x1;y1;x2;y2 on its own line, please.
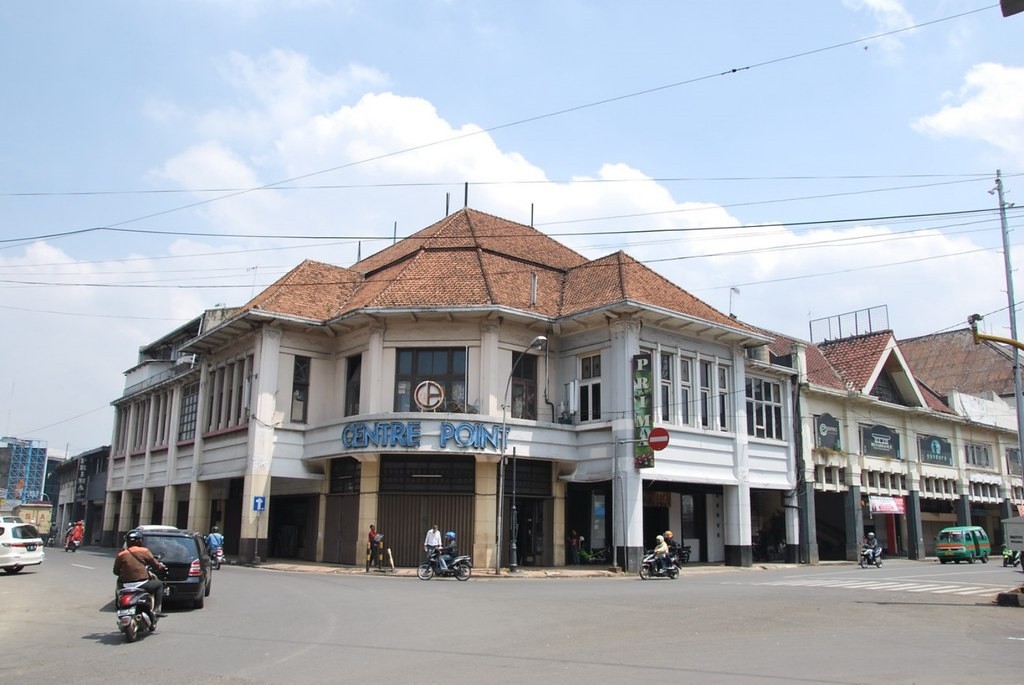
76;521;82;525
127;529;143;539
663;531;673;538
52;522;56;526
212;526;219;533
656;535;664;543
445;532;455;540
868;532;874;538
71;521;76;527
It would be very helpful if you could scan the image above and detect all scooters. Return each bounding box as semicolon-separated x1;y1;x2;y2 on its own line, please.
578;546;612;564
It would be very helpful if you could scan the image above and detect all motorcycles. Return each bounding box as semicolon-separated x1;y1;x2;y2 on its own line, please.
417;546;473;581
859;542;883;568
1001;544;1021;567
47;533;56;547
115;550;168;642
639;548;682;580
202;534;226;570
676;545;692;564
64;528;81;552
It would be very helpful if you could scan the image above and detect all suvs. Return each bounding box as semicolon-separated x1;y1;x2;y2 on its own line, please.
0;522;46;574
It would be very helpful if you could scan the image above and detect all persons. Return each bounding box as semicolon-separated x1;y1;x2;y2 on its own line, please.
112;529;167;617
424;525;458;571
567;530;581;564
203;526;224;560
368;525;379;567
862;532;878;564
47;521;84;549
654;530;678;573
1002;544;1015;558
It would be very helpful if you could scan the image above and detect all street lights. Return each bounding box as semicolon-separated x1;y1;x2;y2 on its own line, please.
494;335;549;576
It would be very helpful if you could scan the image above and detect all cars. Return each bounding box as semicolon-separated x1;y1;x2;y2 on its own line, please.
123;525;181;552
114;529;215;611
0;516;23;523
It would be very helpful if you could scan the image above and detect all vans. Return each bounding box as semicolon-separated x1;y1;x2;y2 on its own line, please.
933;525;992;564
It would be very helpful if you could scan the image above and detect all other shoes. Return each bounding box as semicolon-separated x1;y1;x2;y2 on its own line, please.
659;568;667;573
153;608;169;617
666;566;675;569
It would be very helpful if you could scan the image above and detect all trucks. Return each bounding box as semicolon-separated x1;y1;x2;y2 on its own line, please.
11;503;53;535
